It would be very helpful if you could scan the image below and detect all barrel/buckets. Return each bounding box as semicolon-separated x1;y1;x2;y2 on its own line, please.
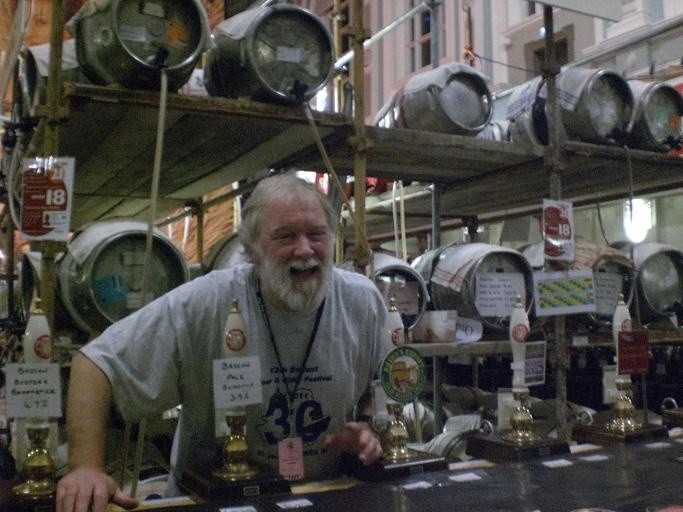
203;4;334;103
506;69;633;147
615;74;683;147
376;65;497;143
76;0;208;93
21;219;683;336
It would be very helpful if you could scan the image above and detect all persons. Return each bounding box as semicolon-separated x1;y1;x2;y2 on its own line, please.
55;174;390;512
